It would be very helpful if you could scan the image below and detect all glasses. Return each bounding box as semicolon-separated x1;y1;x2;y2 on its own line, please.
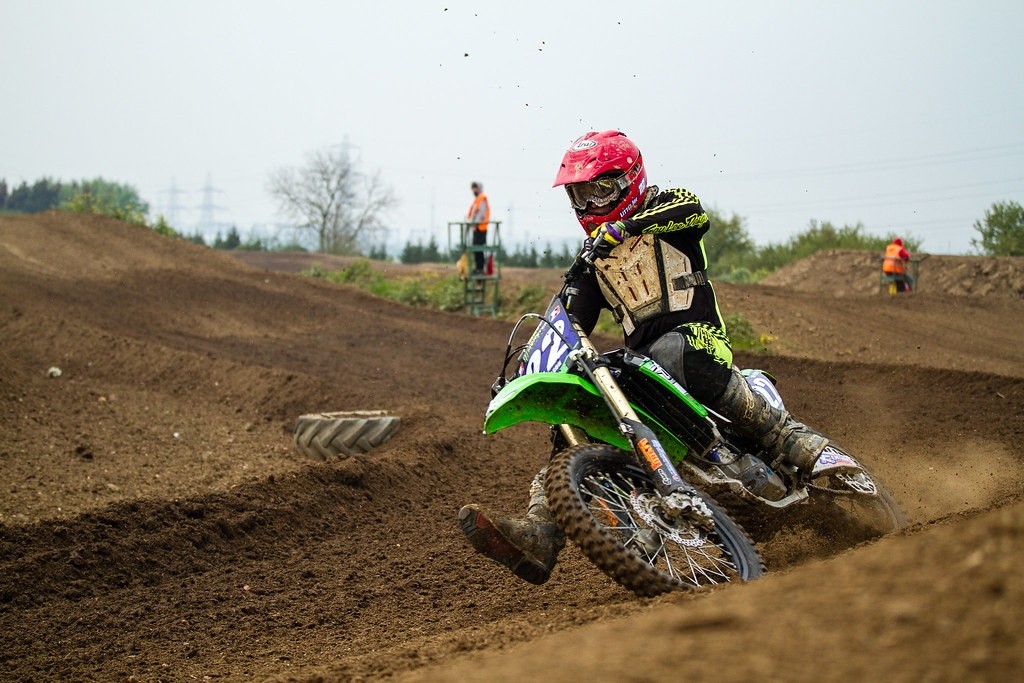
565;149;644;210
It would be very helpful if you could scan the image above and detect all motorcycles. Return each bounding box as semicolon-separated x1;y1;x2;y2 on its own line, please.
483;221;909;599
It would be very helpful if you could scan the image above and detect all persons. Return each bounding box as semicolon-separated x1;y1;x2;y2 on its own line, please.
456;128;830;586
466;181;491;273
883;238;912;294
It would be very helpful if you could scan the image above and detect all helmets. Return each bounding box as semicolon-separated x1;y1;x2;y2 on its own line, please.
551;130;647;236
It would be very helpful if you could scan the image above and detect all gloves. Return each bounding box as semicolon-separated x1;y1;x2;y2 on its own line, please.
585;221;630;259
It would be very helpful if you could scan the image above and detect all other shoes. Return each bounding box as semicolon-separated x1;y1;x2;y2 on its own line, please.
471;269;483;275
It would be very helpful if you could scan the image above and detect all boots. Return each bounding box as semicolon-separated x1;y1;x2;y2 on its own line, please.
713;369;828;472
459;465;564;584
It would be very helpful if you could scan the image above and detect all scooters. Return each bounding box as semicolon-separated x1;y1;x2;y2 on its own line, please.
883;271;913;296
